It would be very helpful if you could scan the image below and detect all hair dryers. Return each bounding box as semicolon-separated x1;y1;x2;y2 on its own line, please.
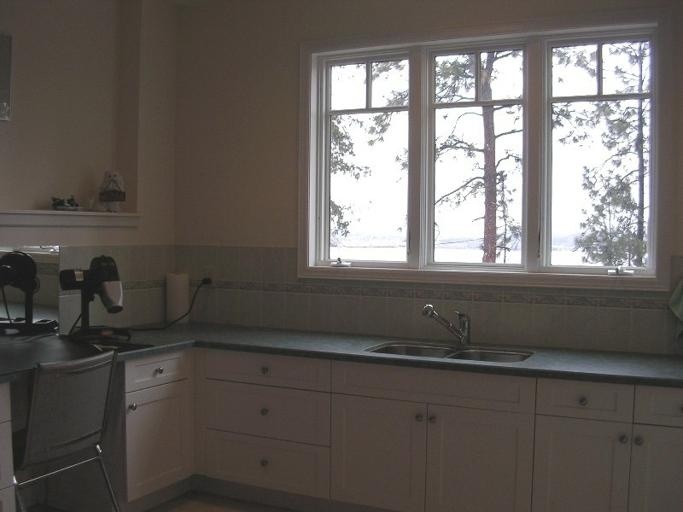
60;256;125;329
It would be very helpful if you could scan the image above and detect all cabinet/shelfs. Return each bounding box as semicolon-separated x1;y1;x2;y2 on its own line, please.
531;377;681;511
194;347;332;512
333;360;536;512
43;347;194;503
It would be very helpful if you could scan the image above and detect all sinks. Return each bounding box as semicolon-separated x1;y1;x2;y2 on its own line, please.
364;341;456;358
447;349;534;363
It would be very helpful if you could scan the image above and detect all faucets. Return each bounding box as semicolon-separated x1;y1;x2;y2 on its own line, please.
421;303;470;349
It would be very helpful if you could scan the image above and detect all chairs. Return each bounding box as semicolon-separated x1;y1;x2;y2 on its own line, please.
12;348;120;512
